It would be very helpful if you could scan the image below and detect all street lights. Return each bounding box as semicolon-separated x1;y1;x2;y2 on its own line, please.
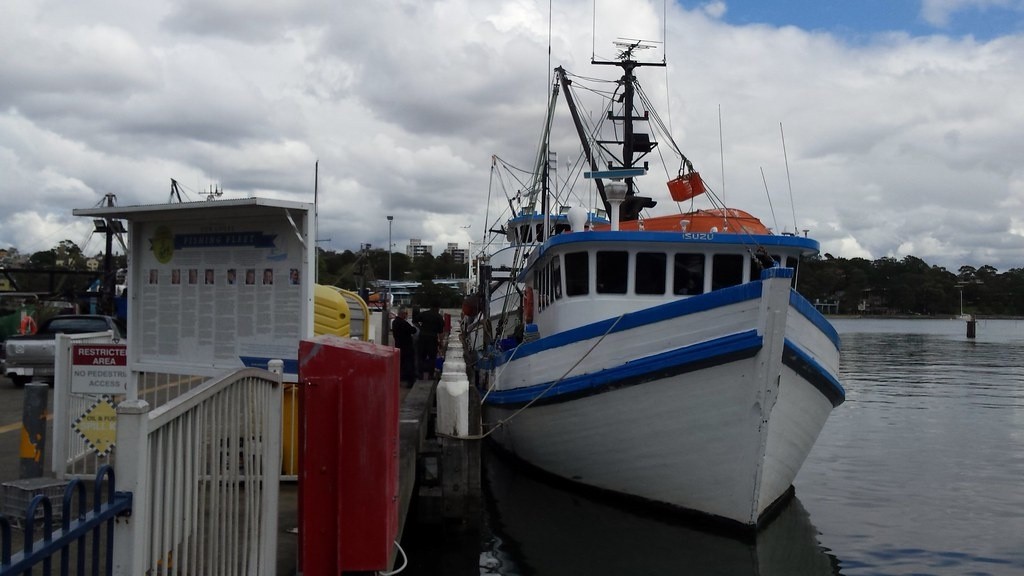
387;215;393;309
92;218;127;297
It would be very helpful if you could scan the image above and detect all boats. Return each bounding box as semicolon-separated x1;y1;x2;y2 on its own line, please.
466;36;846;542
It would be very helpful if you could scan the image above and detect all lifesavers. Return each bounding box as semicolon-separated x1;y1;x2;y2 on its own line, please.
524;287;533;324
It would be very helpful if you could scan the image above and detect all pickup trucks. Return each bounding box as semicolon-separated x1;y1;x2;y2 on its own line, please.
1;308;122;387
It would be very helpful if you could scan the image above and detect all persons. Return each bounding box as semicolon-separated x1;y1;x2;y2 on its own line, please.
149;269;299;285
358;283;446;389
113;287;128;340
